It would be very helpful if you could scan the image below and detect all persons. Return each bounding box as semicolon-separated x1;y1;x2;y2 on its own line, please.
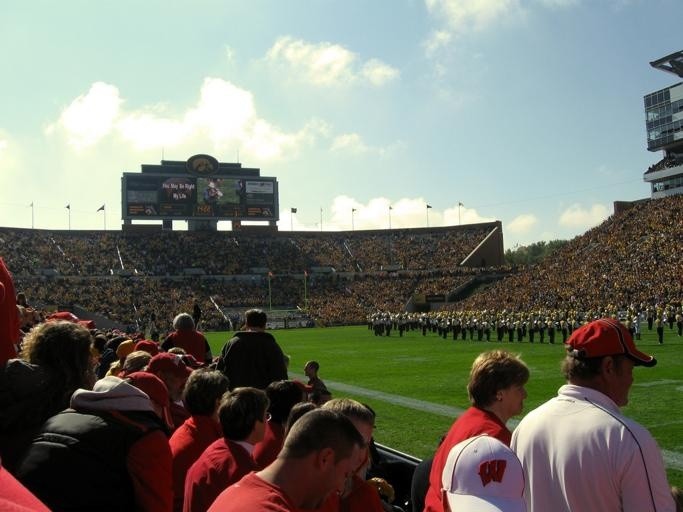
644;155;683;174
0;186;682;511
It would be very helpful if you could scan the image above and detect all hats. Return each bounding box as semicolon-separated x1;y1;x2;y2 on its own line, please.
0;261;18;360
442;434;527;512
48;311;93;327
116;339;204;428
565;319;656;367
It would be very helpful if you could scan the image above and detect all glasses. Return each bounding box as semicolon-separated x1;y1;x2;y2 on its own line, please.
265;417;272;421
